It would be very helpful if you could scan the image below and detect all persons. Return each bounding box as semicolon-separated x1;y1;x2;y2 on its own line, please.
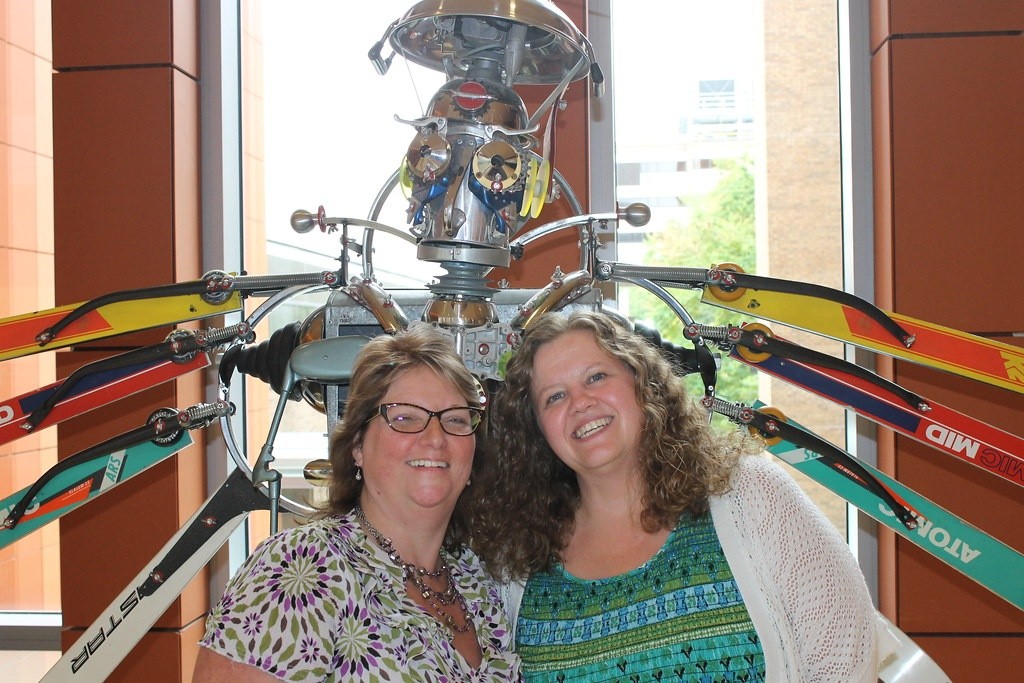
191;321;525;683
444;312;880;683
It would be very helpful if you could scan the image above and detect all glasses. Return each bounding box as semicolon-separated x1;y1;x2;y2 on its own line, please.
360;402;482;437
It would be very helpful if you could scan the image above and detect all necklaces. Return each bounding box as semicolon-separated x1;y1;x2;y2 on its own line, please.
353;501;473;632
